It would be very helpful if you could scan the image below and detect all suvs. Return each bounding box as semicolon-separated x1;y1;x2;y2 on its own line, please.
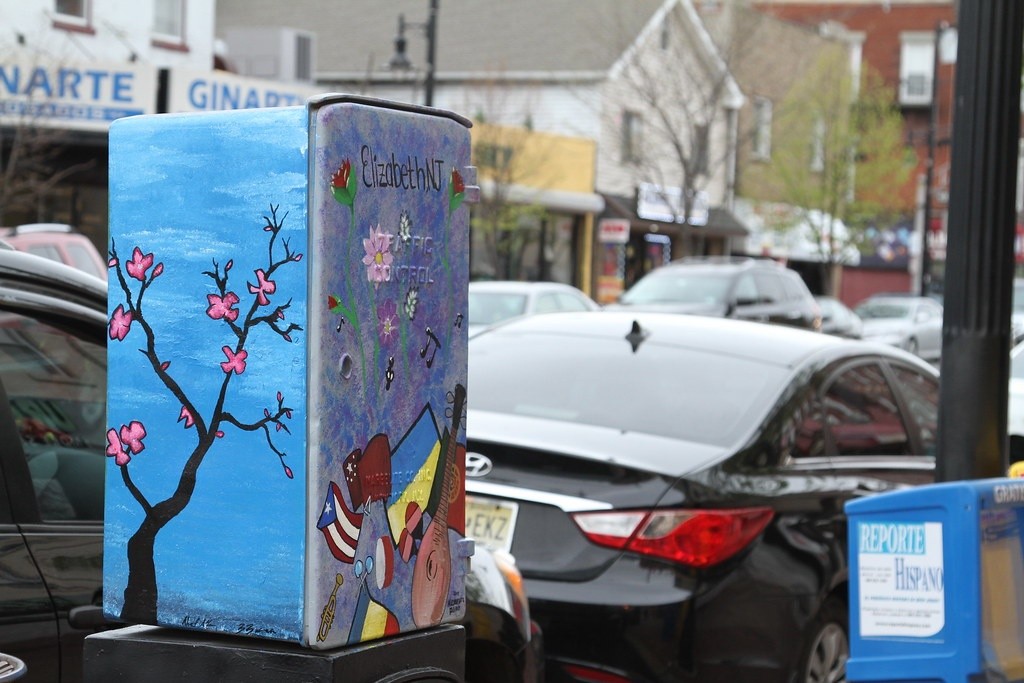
599;253;824;331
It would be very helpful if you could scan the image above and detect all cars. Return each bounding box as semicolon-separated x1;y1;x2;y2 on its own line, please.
465;307;940;682
855;291;942;365
468;276;601;339
815;294;864;342
1;242;545;681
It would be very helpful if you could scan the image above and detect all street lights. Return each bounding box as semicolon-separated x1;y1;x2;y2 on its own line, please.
385;1;441;106
922;18;957;297
